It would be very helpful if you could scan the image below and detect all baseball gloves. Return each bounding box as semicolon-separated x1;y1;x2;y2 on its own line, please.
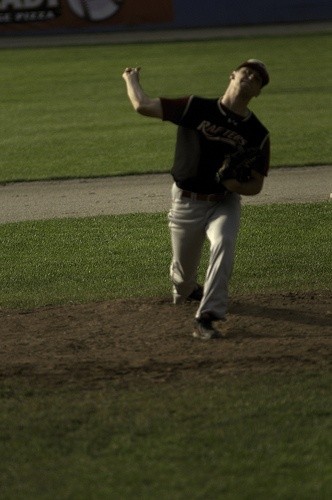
218;154;255;189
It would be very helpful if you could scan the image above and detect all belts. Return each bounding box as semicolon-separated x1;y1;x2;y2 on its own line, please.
182;191;225;204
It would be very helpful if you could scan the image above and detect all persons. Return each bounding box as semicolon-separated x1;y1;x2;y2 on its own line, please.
121;57;271;340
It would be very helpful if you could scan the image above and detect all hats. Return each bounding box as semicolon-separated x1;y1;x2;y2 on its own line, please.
237;59;269;85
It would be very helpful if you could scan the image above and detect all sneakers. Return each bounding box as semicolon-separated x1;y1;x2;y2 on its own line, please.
187;284;204;304
192;316;219;340
